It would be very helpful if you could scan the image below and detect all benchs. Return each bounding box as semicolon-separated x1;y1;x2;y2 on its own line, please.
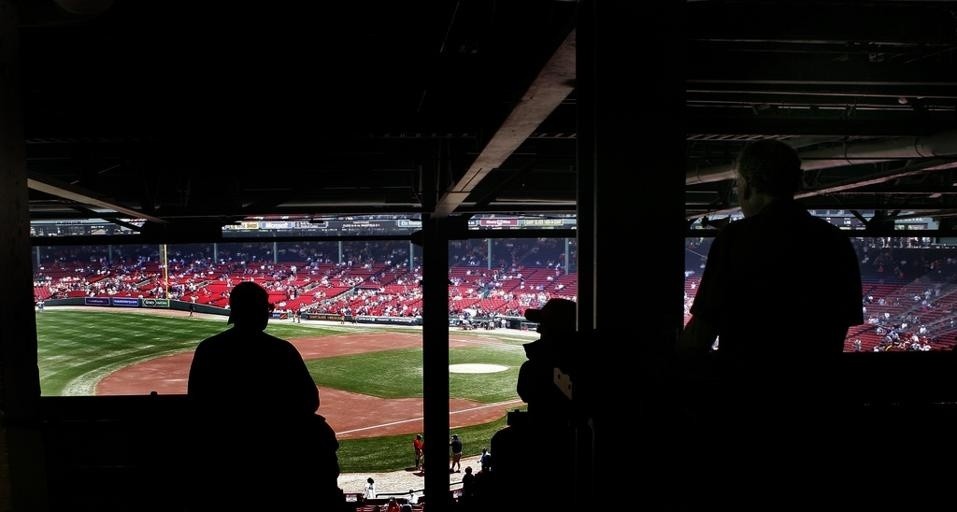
684;239;712;324
843;246;957;352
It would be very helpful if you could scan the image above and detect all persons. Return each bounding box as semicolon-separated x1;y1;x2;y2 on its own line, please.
362;433;493;512
684;139;864;353
188;282;319;413
491;297;575;465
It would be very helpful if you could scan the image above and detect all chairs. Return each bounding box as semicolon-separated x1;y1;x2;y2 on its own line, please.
32;240;577;320
